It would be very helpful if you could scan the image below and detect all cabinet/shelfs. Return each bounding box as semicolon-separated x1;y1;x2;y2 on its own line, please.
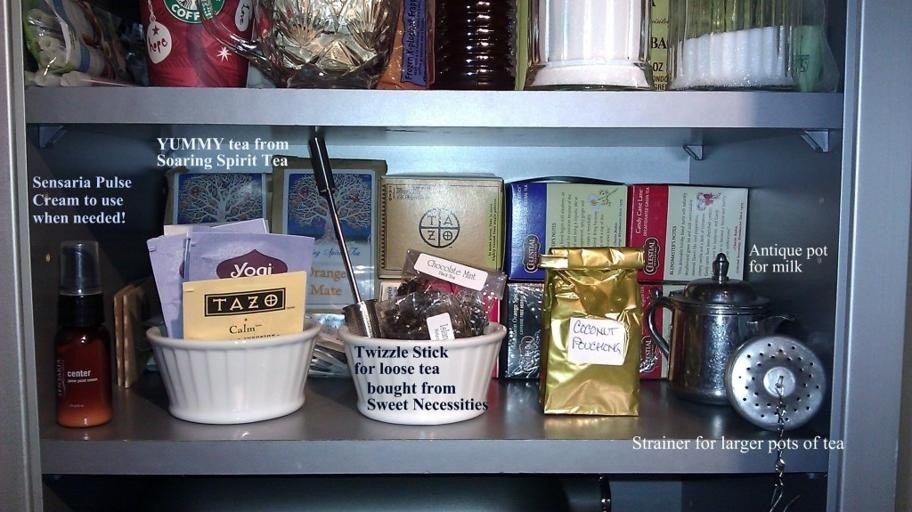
0;0;912;512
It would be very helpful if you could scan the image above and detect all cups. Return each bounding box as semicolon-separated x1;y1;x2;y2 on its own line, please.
143;1;250;86
196;1;399;90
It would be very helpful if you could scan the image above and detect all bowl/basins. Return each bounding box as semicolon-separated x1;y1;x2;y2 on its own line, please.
143;319;327;426
336;321;510;427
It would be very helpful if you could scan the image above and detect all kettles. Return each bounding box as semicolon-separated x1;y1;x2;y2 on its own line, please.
646;254;798;408
